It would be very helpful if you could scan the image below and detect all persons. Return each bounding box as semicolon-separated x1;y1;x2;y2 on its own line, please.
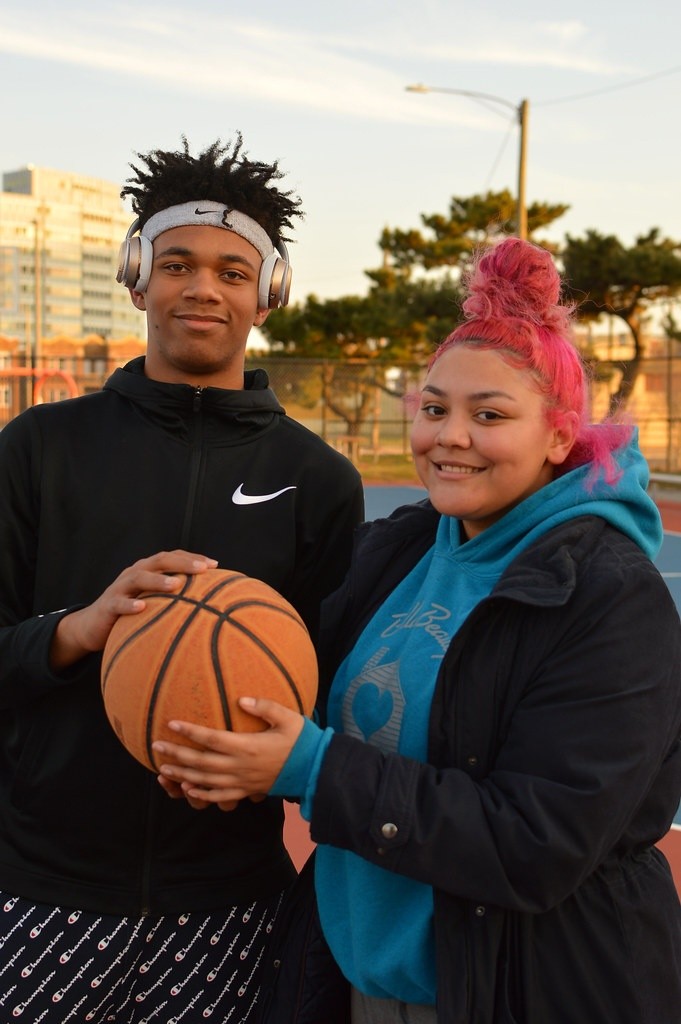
151;237;681;1024
0;131;365;1023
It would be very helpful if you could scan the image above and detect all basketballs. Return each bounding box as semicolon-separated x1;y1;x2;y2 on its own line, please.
99;568;320;775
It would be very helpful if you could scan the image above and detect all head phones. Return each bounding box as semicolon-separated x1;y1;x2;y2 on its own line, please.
115;216;292;309
